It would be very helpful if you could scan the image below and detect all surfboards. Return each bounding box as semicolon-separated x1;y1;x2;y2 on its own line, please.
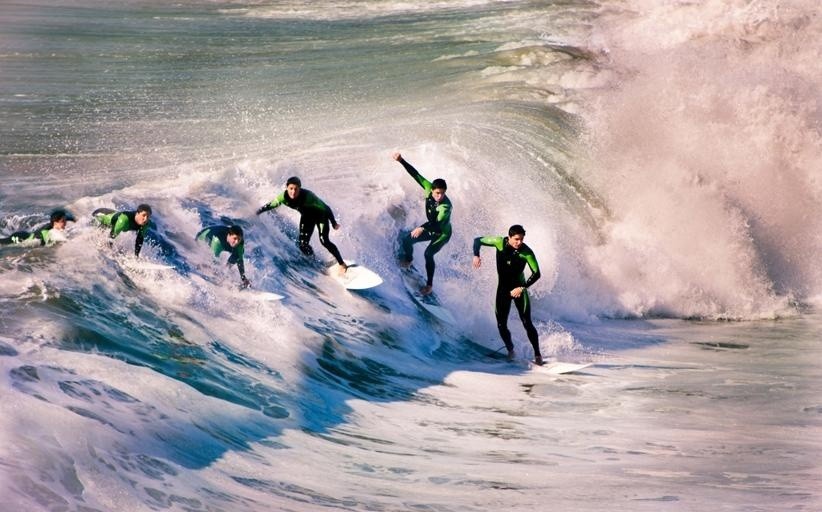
225;280;284;302
392;235;459;328
116;256;177;271
314;251;385;291
466;337;594;374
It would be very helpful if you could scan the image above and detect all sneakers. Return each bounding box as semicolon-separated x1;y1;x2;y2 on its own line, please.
417;285;433;296
506;351;515;363
339;264;349;276
401;259;414;268
535;355;543;365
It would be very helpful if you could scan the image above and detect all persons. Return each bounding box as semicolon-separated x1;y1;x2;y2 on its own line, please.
472;224;542;366
1;210;67;248
91;204;152;259
193;224;251;289
255;176;347;277
391;151;453;295
